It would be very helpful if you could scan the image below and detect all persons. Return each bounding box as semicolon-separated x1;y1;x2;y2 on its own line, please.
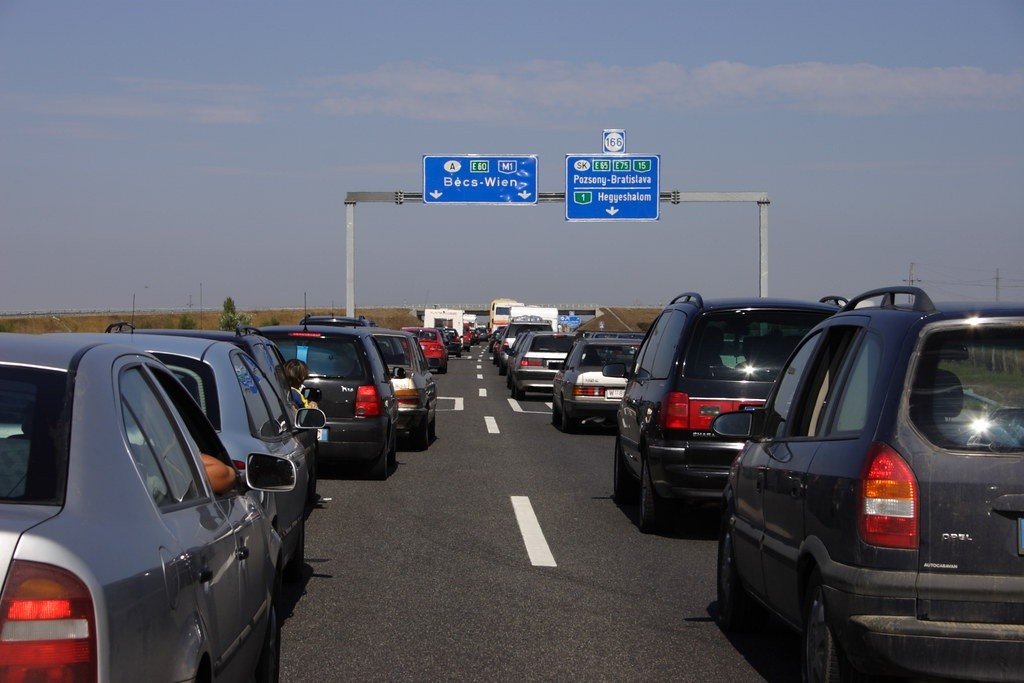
136;445;236;506
282;359;320;411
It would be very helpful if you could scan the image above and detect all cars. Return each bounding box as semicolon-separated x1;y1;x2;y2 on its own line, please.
423;297;649;402
0;331;299;683
49;331;327;631
346;324;441;450
549;331;648;434
399;326;450;375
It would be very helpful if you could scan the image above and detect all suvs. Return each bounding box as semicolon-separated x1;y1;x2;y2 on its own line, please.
248;322;408;482
601;291;853;533
299;314;404;366
712;285;1024;683
114;323;323;513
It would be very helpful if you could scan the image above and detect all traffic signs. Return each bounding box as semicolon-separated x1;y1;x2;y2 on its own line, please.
565;153;660;222
422;155;537;204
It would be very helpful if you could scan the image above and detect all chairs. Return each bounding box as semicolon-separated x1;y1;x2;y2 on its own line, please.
933;370;981;446
